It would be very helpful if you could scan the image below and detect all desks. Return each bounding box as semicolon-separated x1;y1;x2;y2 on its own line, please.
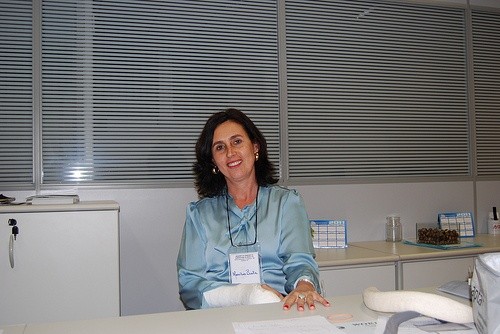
0;284;473;334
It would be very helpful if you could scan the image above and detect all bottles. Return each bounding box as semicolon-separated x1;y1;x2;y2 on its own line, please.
385;216;402;242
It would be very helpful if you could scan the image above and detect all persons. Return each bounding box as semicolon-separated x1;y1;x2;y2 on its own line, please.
176;109;330;311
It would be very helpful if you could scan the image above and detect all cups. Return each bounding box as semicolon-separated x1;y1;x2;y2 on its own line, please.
488;219;500;235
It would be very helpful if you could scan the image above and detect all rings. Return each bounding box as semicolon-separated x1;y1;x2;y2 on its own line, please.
297;295;306;299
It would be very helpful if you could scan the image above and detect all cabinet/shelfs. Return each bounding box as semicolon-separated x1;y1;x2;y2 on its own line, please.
314;234;500;296
0;201;119;320
